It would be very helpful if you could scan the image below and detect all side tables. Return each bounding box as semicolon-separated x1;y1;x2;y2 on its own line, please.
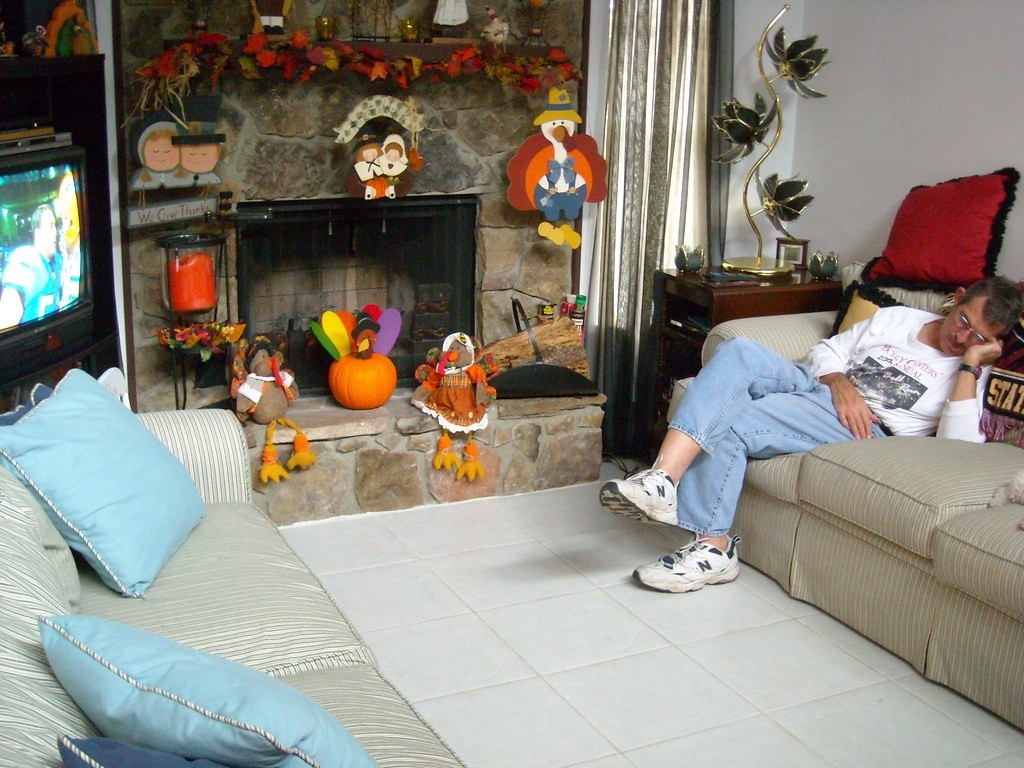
646;271;843;466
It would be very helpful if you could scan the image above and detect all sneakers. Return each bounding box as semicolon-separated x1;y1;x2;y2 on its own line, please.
632;531;741;593
599;454;678;526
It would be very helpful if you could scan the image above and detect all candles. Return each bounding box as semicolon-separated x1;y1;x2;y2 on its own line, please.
170;253;216;309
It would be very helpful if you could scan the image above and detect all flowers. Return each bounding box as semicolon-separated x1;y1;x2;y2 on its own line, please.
158;323;247;361
709;4;832;276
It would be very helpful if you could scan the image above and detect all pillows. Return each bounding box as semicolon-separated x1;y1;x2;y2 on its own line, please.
863;169;1016;290
0;368;203;595
56;733;232;768
835;285;903;337
37;613;378;768
0;383;53;426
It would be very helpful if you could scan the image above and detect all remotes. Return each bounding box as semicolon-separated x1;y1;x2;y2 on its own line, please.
703;272;760;284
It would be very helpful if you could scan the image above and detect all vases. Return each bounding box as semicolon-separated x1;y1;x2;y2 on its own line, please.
197;354;228;389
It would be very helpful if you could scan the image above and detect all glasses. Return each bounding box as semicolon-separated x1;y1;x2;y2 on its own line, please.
955;299;986;345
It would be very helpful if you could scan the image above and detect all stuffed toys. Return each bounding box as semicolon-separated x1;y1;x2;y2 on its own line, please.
410;332;497;481
231;336;317;484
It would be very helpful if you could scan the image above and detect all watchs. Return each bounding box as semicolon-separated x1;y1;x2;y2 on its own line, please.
959;363;983;380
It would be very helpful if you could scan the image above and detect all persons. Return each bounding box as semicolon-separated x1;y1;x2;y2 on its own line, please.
598;274;1024;595
0;202;81;329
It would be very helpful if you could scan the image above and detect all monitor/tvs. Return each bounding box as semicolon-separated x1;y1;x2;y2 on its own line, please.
0;143;94;385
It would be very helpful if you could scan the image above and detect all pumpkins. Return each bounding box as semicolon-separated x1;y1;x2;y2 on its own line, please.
328;352;398;410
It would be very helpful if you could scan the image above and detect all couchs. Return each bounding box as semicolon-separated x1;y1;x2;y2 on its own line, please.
667;284;1024;731
0;408;462;768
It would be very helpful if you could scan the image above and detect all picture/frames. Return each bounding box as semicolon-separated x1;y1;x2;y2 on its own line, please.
776;238;809;268
